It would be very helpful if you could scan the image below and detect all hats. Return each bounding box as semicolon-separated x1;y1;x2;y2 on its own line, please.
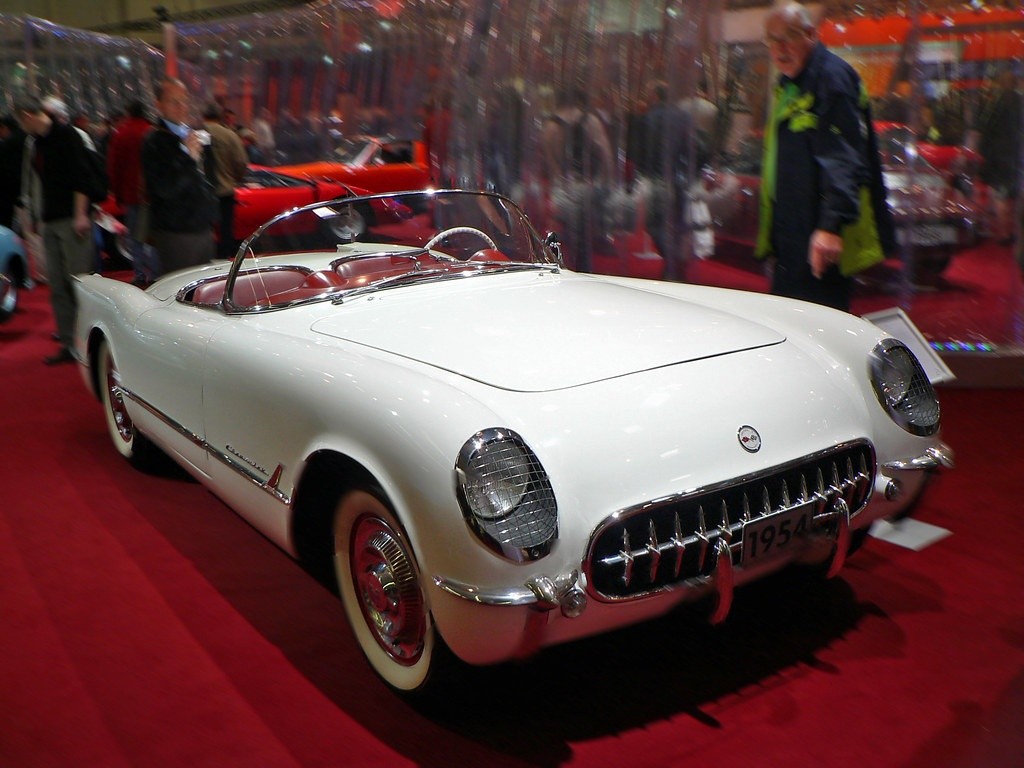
42;98;69;117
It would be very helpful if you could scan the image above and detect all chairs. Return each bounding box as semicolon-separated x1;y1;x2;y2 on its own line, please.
191;266;332;311
329;248;438;291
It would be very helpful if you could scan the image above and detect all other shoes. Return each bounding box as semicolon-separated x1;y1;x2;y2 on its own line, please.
43;350;73;365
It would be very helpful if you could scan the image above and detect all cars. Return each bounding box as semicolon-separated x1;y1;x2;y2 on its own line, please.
687;134;979;290
0;224;35;323
70;187;952;697
877;119;1014;244
95;164;413;267
275;135;433;202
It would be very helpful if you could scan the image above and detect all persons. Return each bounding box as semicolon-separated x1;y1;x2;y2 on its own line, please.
8;95;96;367
105;101;157;246
539;89;625;274
626;81;695;282
0;71;1023;320
754;0;898;313
138;77;217;284
198;101;247;259
